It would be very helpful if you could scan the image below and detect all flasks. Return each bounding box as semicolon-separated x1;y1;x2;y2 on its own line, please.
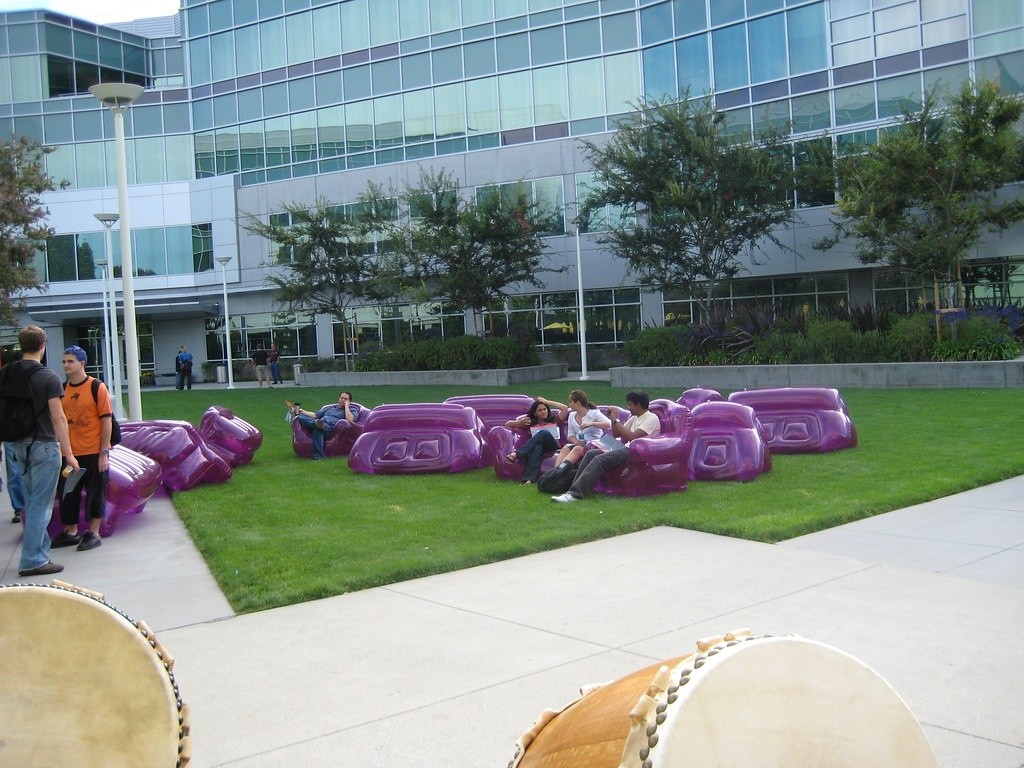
295;402;300;416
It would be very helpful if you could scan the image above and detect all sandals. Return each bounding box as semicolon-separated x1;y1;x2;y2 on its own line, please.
506;453;516;463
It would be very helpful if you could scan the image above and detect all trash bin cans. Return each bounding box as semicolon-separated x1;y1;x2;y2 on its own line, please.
292;364;303;386
216;364;228;384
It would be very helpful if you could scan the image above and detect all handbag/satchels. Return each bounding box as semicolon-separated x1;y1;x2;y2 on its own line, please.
183;360;192;371
536;460;578;494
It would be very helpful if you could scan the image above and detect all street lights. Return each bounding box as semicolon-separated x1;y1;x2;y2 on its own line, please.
566;216;591;381
93;259;116;413
88;82;146;428
214;256;236;389
93;212;128;423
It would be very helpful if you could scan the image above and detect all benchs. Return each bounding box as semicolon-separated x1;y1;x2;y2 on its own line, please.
20;386;858;541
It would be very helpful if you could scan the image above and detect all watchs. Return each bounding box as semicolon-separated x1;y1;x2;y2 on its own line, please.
614;419;620;425
101;448;109;455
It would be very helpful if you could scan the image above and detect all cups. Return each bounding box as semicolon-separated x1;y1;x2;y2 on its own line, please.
62;464;74;477
525;417;531;426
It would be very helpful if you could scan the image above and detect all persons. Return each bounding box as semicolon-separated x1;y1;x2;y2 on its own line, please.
555;390;612;479
290;392;359;461
551;391;661;503
0;325;79;576
505;397;568;486
252;343;283;388
175;346;193;390
50;345;112;550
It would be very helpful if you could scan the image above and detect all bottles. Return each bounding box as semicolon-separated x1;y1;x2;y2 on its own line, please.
578;429;585;441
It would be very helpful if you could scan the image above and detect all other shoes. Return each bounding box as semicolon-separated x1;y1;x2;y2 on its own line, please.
12;510;21;522
315;419;327;432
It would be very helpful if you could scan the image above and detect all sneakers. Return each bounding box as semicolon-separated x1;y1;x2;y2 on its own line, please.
51;531;81;548
20;562;64;575
551;494;577;503
77;530;101;551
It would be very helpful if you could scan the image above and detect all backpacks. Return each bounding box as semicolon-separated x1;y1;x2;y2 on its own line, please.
63;378;122;446
